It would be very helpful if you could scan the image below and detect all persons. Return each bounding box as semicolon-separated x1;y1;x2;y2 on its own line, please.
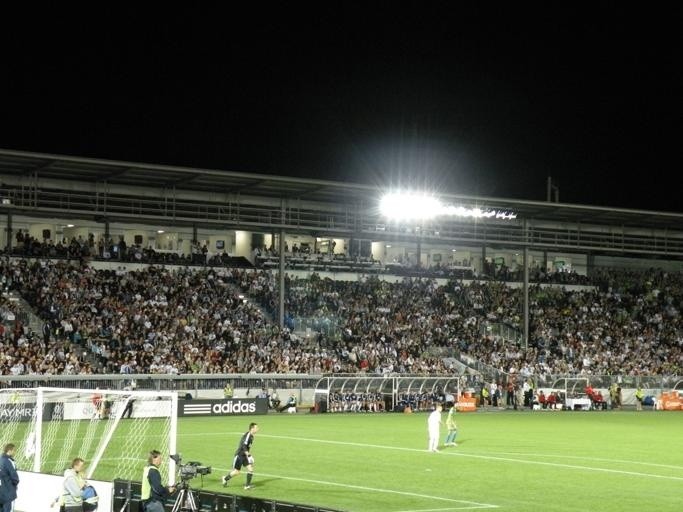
427;404;444;451
101;400;113;418
60;457;87;512
89;394;101;420
443;401;460;447
221;421;258;489
0;442;20;511
120;399;134;419
80;482;98;511
139;450;175;512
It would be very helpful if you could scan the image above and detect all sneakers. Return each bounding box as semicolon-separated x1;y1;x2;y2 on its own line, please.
222;475;256;491
